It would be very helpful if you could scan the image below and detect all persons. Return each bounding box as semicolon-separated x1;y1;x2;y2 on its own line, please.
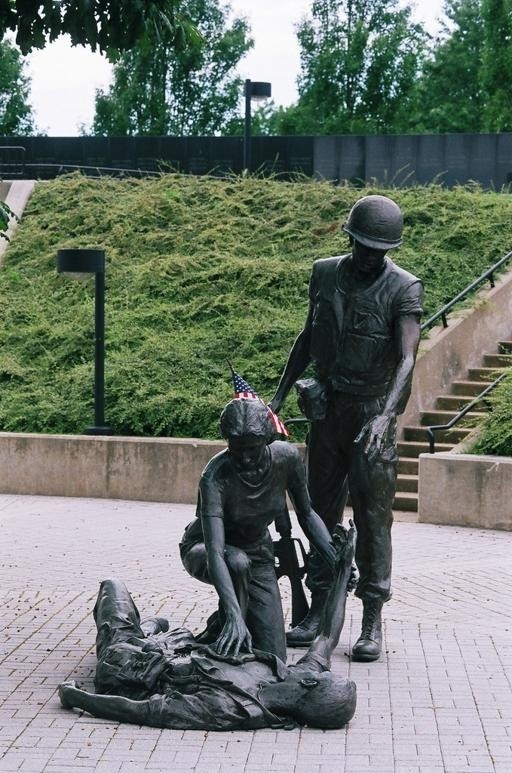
178;396;357;666
263;195;428;658
57;518;362;734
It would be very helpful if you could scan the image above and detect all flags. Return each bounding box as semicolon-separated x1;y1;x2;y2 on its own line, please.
232;365;290;437
